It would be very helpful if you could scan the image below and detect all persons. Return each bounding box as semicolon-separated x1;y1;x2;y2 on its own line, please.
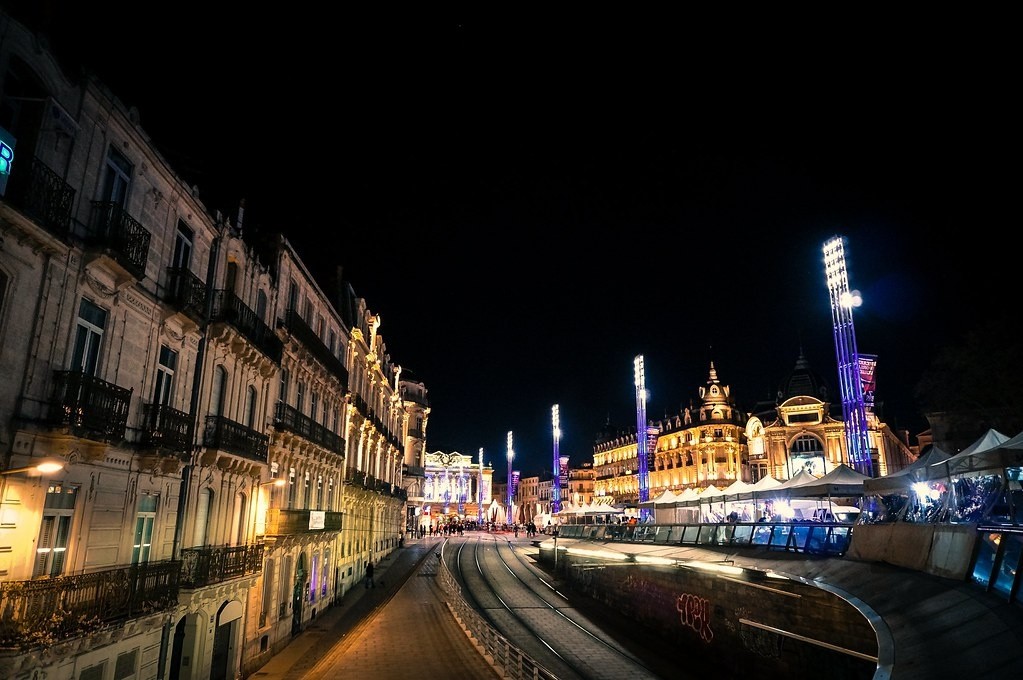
572;509;822;552
405;516;550;538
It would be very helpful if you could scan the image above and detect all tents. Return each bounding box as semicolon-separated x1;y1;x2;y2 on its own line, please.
555;429;1023;526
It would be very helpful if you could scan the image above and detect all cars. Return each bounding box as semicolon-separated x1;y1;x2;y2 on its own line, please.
812;505;863;523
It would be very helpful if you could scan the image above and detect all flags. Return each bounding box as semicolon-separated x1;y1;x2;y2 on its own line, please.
511;471;520;497
844;353;877;407
559;455;570;488
